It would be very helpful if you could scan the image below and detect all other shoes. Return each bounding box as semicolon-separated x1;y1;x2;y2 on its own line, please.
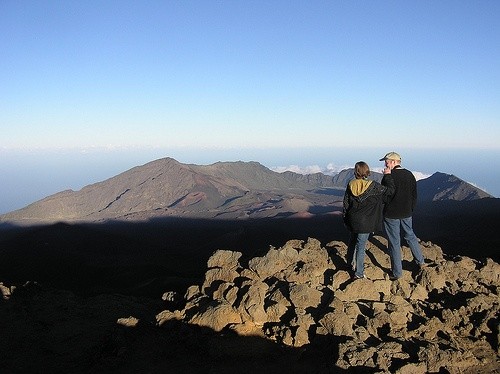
353;275;364;279
418;262;429;269
344;266;356;272
389;275;398;280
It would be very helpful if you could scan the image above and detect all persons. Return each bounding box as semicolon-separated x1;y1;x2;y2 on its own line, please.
342;161;396;280
379;151;424;280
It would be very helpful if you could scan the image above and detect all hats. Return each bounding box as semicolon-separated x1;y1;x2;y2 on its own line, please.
380;152;401;161
